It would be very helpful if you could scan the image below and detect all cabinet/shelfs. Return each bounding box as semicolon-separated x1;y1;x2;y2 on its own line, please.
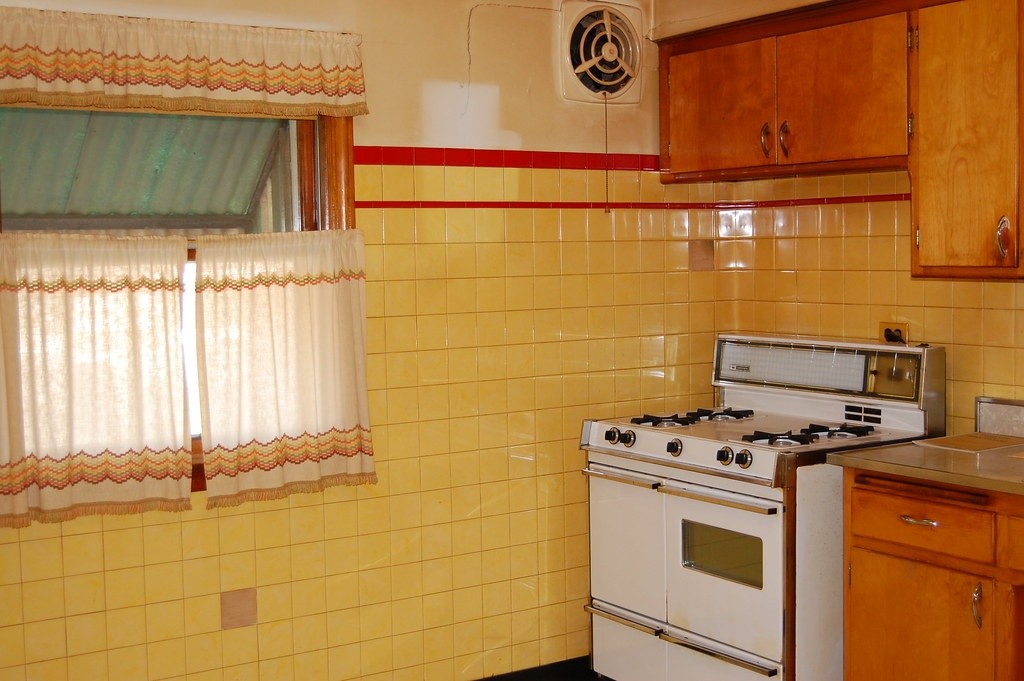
657;0;909;185
843;466;1024;681
910;0;1024;280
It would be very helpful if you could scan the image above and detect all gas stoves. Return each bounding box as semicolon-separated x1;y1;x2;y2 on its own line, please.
587;397;923;481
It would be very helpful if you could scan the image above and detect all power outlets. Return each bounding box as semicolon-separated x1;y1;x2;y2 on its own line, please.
879;322;909;346
